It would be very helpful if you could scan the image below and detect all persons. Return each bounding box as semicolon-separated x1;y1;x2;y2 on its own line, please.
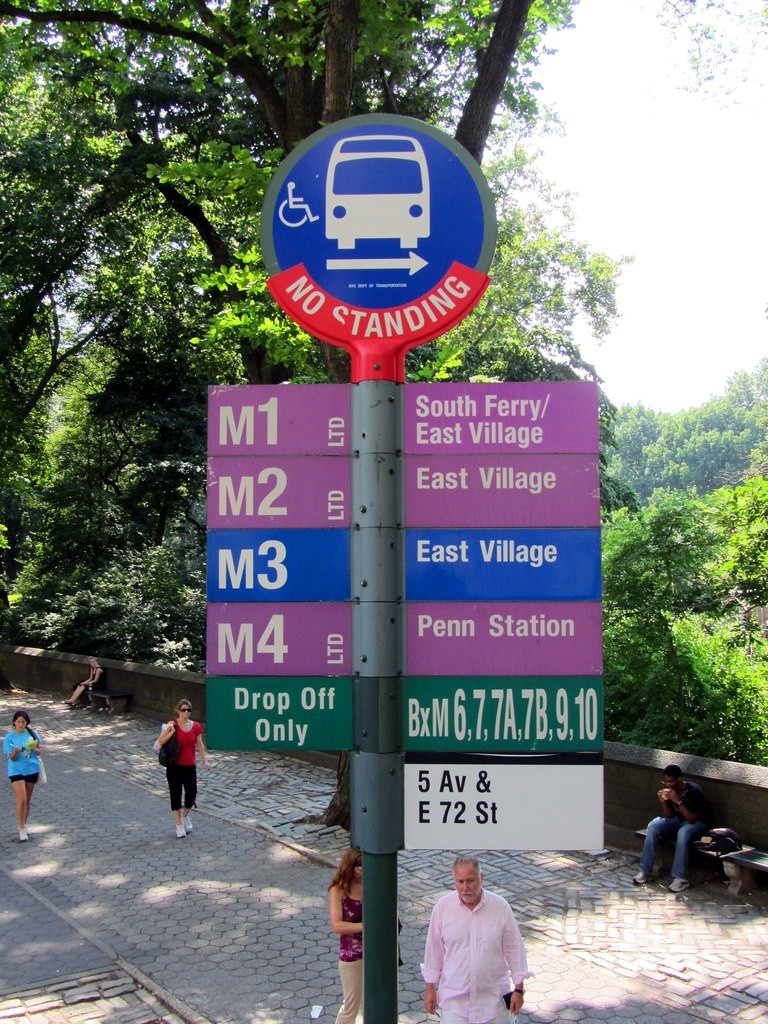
66;657;104;707
158;699;211;838
328;847;404;1024
420;857;534;1024
632;765;714;893
3;709;41;842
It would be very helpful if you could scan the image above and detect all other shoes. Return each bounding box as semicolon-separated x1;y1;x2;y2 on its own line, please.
65;700;76;705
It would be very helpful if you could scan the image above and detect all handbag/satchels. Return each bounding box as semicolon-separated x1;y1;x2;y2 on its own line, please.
37;755;47;786
157;723;179;766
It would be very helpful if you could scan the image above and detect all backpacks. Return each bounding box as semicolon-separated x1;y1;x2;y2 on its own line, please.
694;827;742;854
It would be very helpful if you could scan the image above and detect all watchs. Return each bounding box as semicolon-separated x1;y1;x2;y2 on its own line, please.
678;800;683;806
514;988;525;994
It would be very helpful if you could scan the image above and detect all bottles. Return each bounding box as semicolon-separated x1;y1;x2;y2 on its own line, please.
89;681;92;691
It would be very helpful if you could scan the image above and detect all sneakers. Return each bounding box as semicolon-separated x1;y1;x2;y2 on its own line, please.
668;877;689;892
632;872;654;884
176;815;193;837
19;824;30;840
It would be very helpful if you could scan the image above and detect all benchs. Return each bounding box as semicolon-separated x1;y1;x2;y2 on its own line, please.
635;828;768;895
71;688;129;715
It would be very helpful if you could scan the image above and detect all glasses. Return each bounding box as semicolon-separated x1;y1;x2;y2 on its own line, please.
662;780;677;787
180;708;192;713
